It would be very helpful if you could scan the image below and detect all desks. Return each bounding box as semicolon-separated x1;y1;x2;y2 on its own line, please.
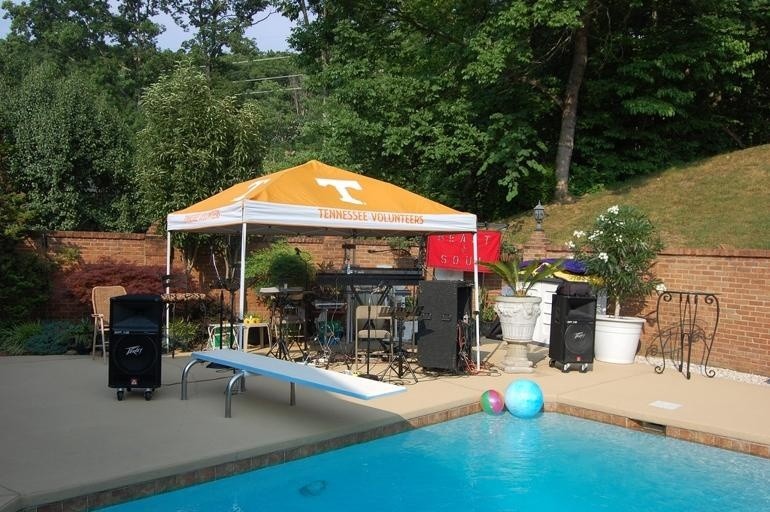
654;289;719;377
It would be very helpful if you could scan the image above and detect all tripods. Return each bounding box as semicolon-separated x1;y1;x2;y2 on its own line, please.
264;289;418;385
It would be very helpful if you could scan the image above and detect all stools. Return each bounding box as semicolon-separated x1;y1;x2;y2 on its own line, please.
241;322;273;351
207;324;241;350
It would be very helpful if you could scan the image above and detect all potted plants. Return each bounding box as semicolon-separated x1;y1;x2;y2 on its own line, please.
475;252;566;344
564;202;667;367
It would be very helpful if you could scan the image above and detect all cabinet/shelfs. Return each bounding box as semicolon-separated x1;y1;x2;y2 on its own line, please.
501;277;606;343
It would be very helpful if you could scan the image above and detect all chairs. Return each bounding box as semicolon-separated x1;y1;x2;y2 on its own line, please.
355;303;393;376
162;274;210;359
90;283;129;361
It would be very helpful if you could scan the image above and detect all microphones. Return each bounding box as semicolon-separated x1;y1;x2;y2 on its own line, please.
295;247;302;253
368;250;376;253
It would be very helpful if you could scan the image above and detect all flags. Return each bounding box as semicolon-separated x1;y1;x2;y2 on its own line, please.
424;228;503;275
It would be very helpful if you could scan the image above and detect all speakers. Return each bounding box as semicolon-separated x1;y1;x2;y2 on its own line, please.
549;293;596;364
108;294;162;388
